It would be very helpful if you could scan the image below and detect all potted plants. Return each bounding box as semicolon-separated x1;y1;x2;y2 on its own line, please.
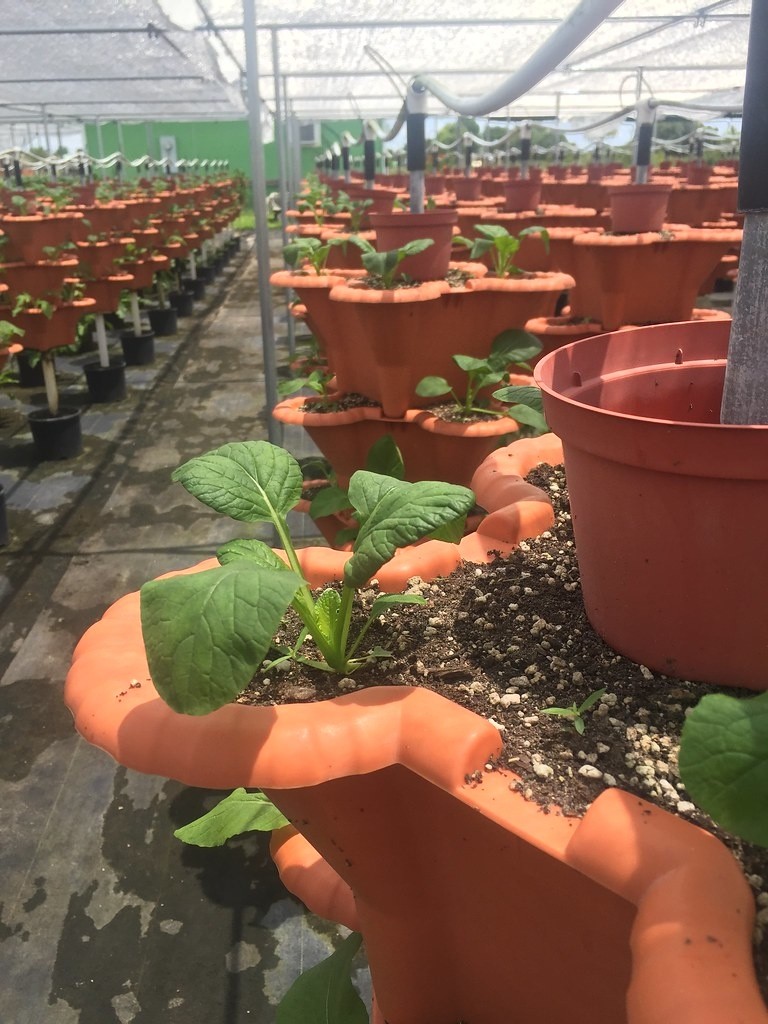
66;432;768;1024
0;171;241;368
265;173;742;396
267;224;575;421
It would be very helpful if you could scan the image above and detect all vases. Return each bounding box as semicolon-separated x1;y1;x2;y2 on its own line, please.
120;232;247;369
27;406;83;461
83;361;127;404
319;159;744;278
18;352;57;389
534;319;768;696
